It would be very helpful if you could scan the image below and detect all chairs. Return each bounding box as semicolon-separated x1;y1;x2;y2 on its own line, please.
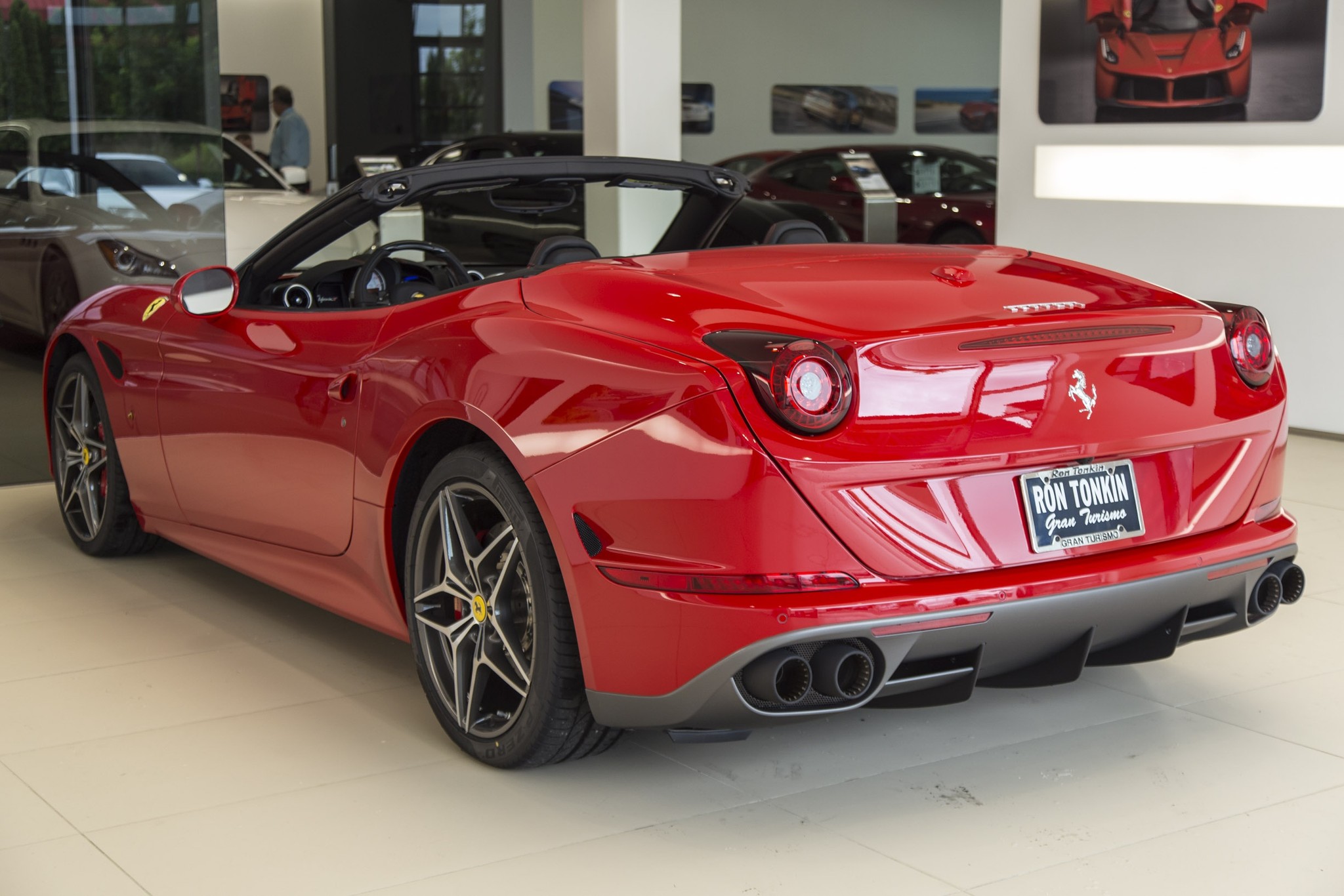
761;218;828;246
525;234;601;269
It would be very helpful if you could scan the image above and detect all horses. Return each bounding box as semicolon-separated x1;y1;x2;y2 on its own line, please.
1068;368;1097;420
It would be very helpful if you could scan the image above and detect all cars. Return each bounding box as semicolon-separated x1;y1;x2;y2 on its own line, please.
550;84;585;132
679;93;714;123
0;116;381;338
1085;0;1268;108
799;84;864;128
710;151;801;185
738;143;998;250
960;96;998;133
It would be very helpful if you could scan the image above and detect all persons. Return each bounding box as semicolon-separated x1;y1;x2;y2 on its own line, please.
236;134;271;165
271;86;311;195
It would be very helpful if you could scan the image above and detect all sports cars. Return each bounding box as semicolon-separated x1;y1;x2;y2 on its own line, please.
44;154;1310;767
